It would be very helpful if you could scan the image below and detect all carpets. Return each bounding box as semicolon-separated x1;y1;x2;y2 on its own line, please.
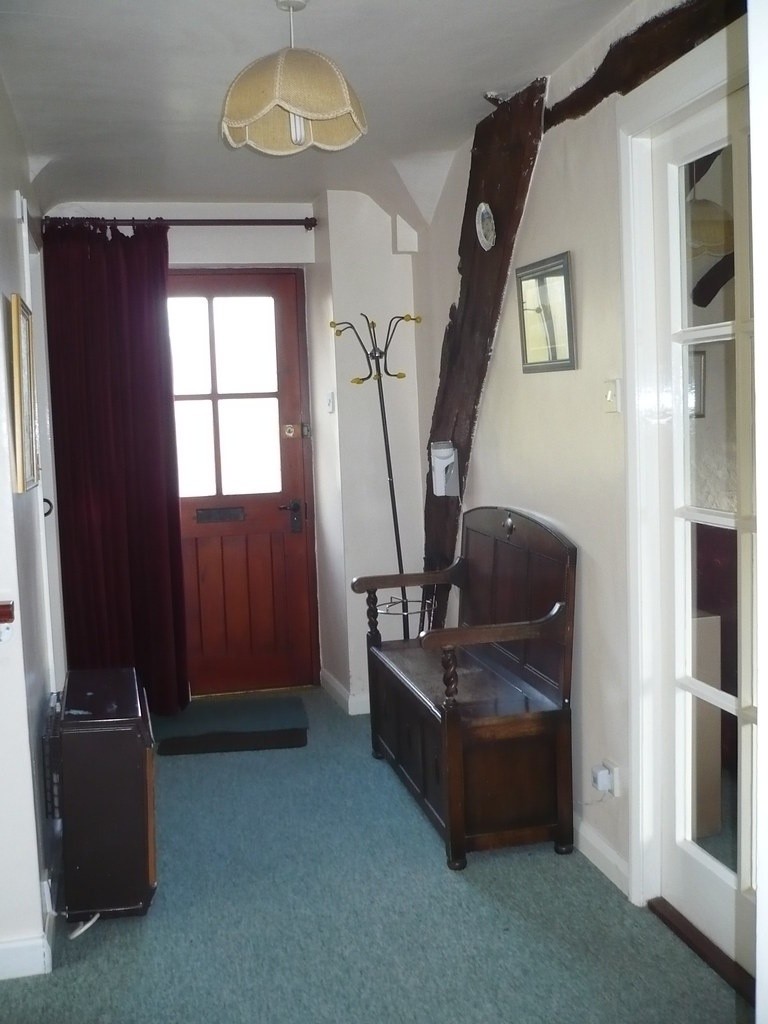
156;728;307;756
149;694;309;739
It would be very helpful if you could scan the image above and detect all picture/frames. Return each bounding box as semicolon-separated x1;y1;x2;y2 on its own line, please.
10;290;39;494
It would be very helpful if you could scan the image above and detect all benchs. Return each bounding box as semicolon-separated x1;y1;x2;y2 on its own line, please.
349;506;578;870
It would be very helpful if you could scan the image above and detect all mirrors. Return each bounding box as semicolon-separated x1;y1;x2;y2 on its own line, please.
512;250;578;375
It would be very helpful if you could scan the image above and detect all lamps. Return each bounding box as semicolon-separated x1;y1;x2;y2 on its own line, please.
220;0;369;156
